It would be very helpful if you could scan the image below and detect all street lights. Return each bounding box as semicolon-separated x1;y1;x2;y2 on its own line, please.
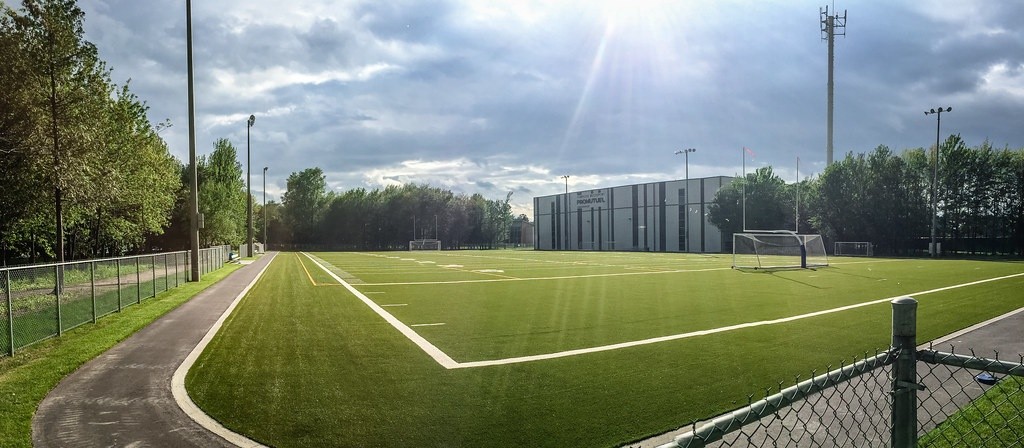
561;175;569;251
264;167;268;252
924;107;952;259
675;148;696;254
247;115;255;258
